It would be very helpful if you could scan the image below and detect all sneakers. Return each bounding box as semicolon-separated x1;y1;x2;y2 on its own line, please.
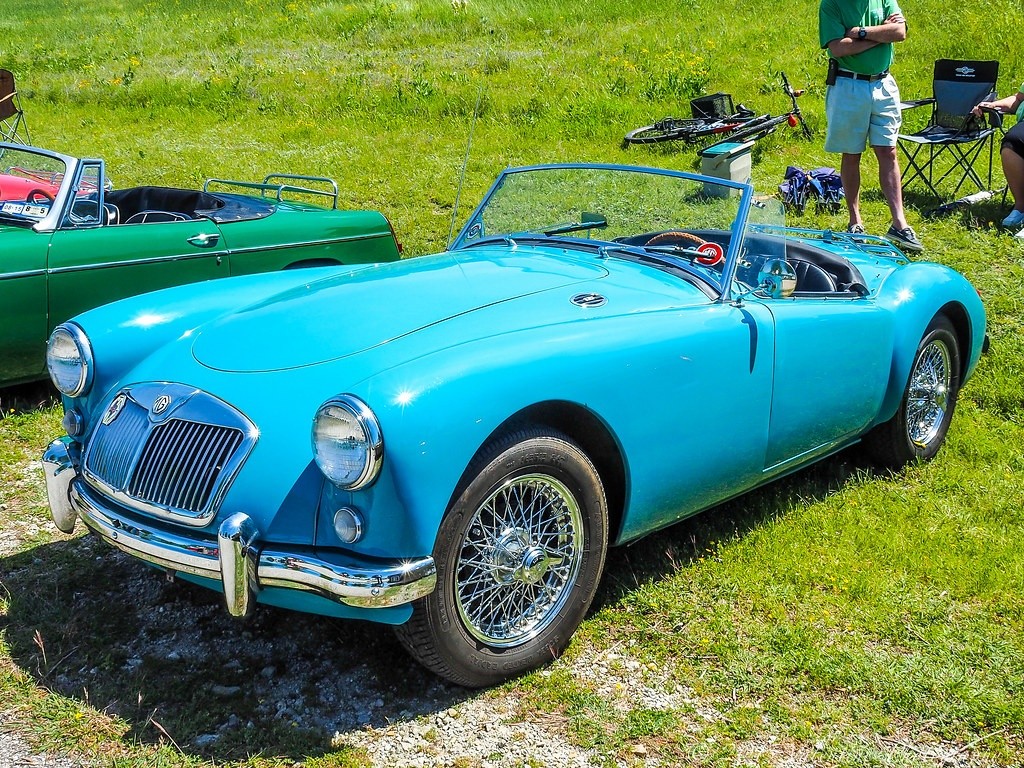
848;222;867;244
885;225;925;252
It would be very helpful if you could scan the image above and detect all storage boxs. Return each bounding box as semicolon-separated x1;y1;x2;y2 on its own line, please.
702;141;757;198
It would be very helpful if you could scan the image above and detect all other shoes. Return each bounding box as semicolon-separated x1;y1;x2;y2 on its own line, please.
1014;229;1024;239
1002;210;1024;228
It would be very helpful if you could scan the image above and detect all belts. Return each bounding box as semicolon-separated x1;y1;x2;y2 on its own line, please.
836;67;890;83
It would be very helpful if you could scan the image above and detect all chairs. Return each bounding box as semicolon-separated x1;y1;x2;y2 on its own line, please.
897;59;1000;205
739;254;838;292
0;69;32;157
980;106;1016;213
73;200;120;226
124;210;191;224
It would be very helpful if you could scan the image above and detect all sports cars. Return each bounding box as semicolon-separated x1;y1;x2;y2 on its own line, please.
0;141;401;391
47;162;992;692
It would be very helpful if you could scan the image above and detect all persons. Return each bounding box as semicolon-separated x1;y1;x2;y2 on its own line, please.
819;0;925;251
972;83;1024;239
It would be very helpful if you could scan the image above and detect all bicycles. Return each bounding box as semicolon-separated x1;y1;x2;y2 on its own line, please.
623;71;813;156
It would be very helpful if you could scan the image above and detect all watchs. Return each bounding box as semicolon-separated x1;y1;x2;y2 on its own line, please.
858;26;868;40
